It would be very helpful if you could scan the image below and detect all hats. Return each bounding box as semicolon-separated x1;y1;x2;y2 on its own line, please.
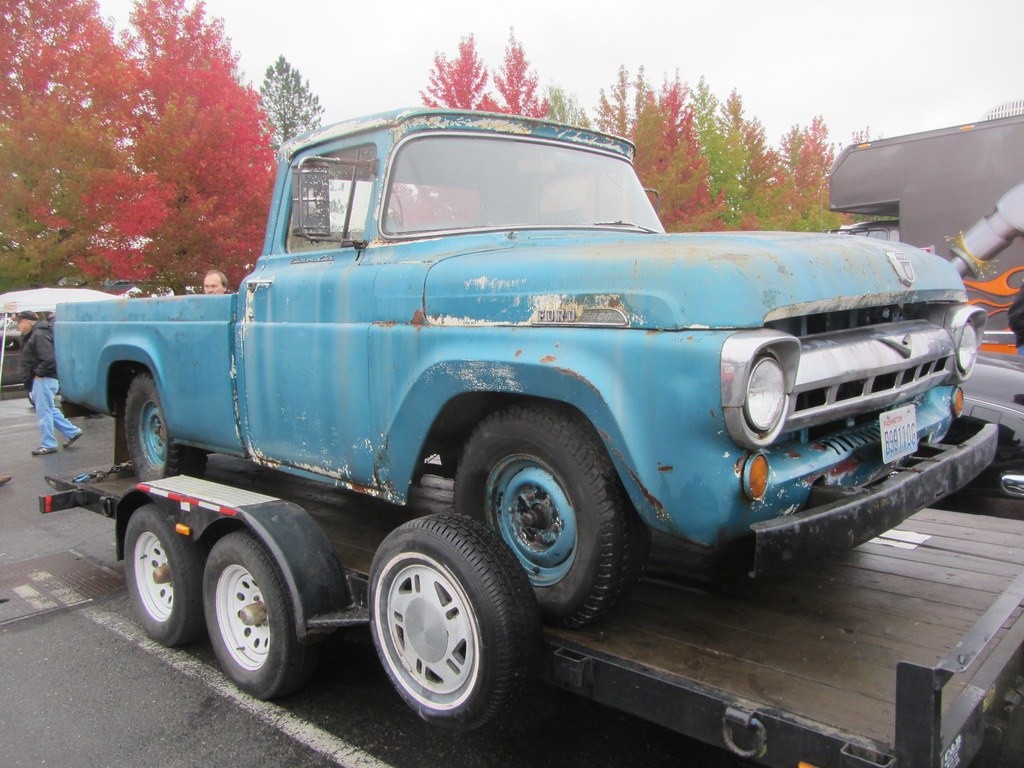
11;311;39;321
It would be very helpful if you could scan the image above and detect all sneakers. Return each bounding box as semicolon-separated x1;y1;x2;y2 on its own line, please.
32;447;57;455
62;431;83;448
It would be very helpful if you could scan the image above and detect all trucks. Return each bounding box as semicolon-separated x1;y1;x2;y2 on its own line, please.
812;113;1023;502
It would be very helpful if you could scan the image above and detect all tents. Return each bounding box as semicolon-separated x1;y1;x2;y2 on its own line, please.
0;288;116;385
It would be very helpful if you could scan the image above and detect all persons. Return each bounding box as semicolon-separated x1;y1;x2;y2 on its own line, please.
13;310;84;454
204;270;231;295
1008;282;1024;360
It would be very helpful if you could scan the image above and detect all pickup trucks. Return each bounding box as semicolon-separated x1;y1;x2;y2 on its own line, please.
48;107;995;626
